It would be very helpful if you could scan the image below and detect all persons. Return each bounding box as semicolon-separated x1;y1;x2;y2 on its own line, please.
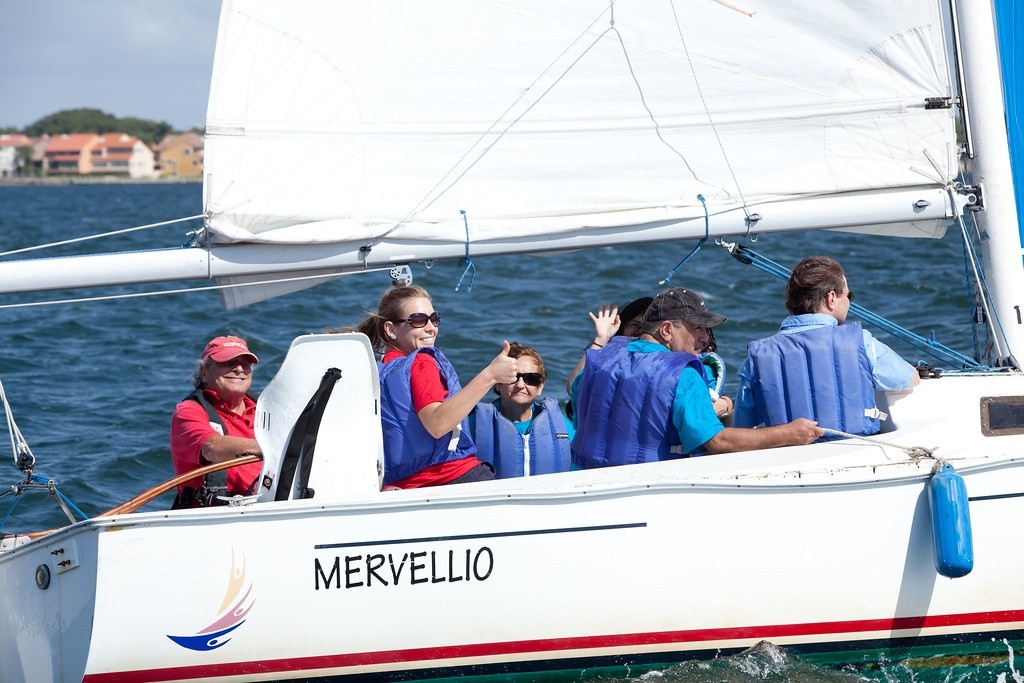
572;287;825;474
170;336;263;507
700;328;733;428
330;286;518;490
732;256;920;442
567;297;654;397
470;342;581;477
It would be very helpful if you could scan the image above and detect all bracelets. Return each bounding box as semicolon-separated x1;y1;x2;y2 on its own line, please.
593;342;602;347
718;396;733;418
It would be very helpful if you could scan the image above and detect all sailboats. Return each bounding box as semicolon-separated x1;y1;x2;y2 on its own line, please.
1;0;1024;683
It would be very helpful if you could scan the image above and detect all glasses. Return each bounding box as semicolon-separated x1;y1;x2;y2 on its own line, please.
835;291;855;303
391;311;442;328
512;372;546;386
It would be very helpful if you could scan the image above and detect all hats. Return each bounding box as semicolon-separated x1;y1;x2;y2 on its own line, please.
643;286;727;328
200;334;260;365
